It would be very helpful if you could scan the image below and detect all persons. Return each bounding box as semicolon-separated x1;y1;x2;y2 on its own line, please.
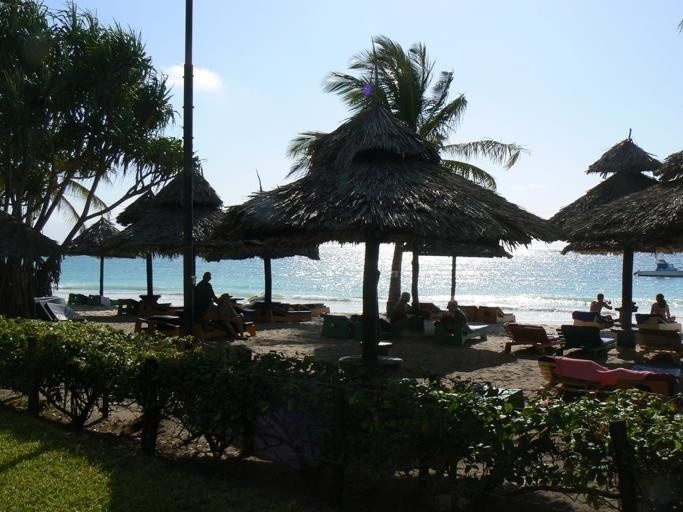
651;294;672;321
590;294;612;320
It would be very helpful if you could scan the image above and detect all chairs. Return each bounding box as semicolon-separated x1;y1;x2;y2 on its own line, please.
505;311;683;394
199;302;362;339
118;295;184;336
381;302;515;363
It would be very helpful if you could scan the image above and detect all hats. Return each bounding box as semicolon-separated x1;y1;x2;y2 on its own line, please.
218;294;232;300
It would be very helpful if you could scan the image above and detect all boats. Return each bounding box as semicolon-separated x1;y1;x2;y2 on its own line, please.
633;259;681;280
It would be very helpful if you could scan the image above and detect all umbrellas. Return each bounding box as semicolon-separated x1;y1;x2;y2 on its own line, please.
565;149;683;253
548;139;663;327
